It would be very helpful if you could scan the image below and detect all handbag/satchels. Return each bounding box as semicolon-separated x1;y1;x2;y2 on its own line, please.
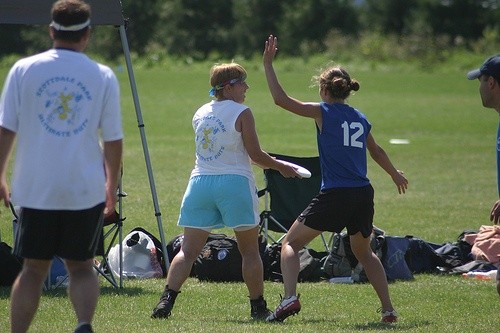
266;240;322;282
0;241;22;286
117;225;167;279
382;224;500;281
168;231;226;278
198;237;274;282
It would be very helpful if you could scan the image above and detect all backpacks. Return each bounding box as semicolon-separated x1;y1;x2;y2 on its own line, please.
325;223;385;282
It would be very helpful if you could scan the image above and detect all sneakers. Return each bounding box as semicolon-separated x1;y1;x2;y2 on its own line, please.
151;286;181;319
376;307;398;323
249;296;273;319
265;293;301;322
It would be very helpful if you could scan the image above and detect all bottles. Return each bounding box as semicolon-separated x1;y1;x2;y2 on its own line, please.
462;272;490;280
326;277;354;284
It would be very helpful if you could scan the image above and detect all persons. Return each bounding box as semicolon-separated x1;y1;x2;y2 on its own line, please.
0;0;124;333
260;34;409;321
466;53;500;297
151;60;302;320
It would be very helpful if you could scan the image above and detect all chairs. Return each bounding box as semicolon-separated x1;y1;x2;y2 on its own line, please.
9;162;128;294
258;153;340;252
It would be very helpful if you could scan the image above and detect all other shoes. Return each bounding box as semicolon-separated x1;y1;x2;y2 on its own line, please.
75;323;94;333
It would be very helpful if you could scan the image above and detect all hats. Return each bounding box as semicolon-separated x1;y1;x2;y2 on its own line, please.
466;55;500;81
108;244;121;280
